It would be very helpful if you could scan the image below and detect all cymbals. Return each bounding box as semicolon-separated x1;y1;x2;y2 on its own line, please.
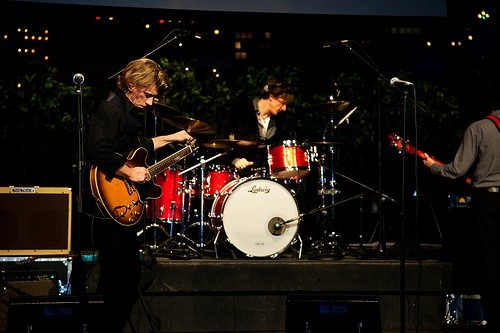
205;139;258;148
312;101;350;112
310;141;342;145
152;101;185;116
164;116;215;134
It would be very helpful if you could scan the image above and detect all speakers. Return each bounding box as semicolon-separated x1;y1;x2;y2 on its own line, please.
0;185;73;255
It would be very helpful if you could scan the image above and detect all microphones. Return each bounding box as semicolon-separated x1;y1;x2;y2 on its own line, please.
274;221;282;229
389;77;413;87
334;107;358;129
72;73;84;85
175;29;202;37
321;40;352;48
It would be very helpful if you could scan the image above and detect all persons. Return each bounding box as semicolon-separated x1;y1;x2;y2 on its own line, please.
422;81;500;248
234;77;297;143
87;57;198;333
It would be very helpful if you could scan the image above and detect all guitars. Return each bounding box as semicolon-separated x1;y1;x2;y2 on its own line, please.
90;138;196;227
388;132;472;185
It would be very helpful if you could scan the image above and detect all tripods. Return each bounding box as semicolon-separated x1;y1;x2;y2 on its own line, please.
135;128;235;259
302;103;362;260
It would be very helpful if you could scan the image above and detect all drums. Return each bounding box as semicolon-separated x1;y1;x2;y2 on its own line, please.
202;165;240;199
210;176;301;259
265;140;312;180
144;164;186;223
